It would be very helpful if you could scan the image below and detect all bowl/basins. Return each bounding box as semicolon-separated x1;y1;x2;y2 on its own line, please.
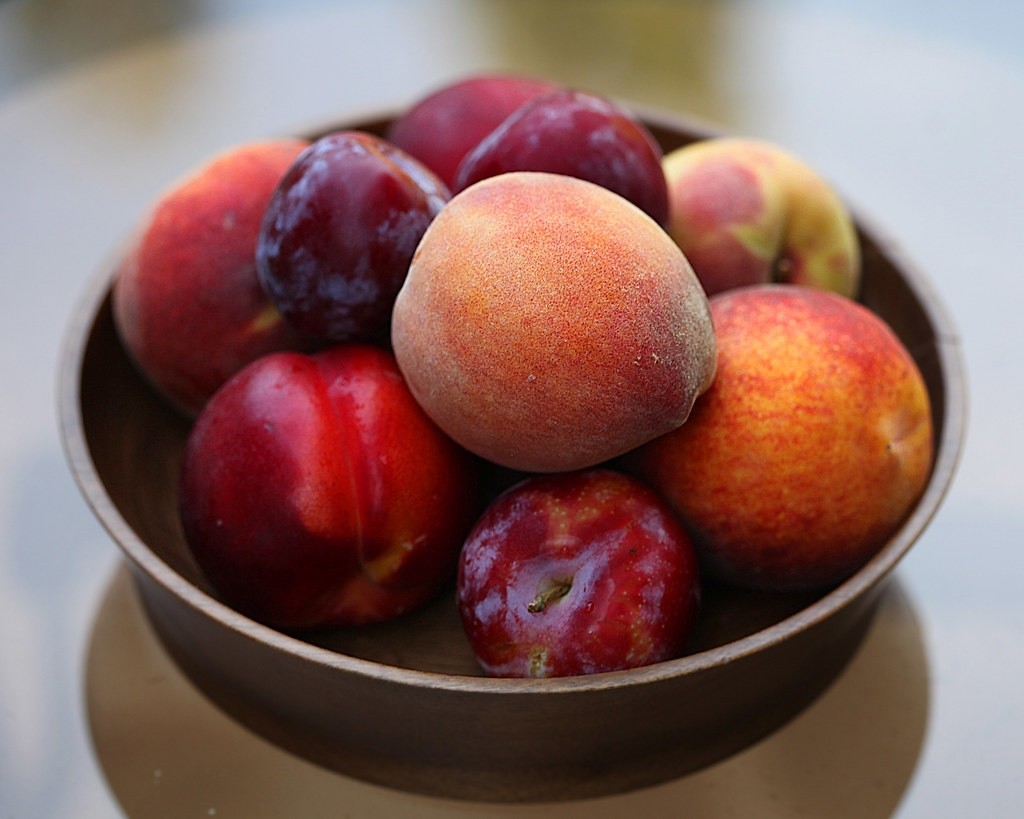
51;105;967;813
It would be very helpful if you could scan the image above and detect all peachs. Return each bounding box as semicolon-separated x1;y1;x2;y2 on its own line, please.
113;82;936;679
